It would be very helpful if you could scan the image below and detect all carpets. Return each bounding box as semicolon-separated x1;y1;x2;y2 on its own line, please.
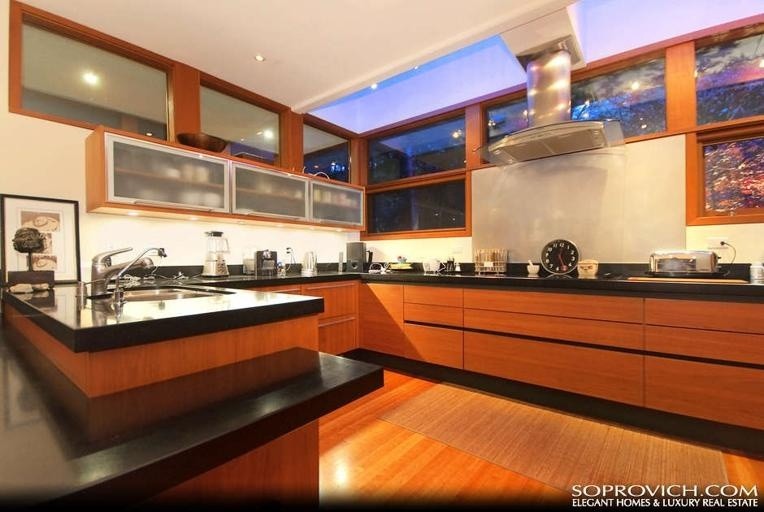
376;383;731;509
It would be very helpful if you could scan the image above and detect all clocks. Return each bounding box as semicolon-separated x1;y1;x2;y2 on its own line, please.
540;239;579;279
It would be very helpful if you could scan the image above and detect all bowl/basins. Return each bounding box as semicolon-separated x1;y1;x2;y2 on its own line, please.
176;133;229;154
577;260;598;276
526;265;540;273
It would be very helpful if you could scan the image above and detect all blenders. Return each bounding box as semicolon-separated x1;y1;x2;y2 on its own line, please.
201;230;230;278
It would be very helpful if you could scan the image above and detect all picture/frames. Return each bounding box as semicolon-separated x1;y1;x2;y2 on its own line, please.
0;194;82;288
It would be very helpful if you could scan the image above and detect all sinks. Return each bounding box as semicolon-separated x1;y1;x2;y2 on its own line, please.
124;283;206;295
120;292;213;302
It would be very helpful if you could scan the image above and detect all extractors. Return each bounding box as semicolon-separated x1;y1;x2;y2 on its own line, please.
473;35;626;169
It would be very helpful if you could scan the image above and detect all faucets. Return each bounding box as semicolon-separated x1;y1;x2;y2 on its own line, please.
110;245;168;306
90;246;157;297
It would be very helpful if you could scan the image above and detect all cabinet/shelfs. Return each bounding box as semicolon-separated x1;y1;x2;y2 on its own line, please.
643;291;763;433
302;278;358;355
404;282;464;369
84;126;231;220
358;280;404;359
463;282;644;408
309;174;368;231
230;156;310;225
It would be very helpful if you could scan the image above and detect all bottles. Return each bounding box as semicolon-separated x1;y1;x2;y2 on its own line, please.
454;262;461;272
749;262;764;285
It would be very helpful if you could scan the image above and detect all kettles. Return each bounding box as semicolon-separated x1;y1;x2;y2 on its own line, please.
301;250;317;273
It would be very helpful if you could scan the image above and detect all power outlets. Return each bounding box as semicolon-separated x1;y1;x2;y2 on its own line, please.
705;237;729;249
